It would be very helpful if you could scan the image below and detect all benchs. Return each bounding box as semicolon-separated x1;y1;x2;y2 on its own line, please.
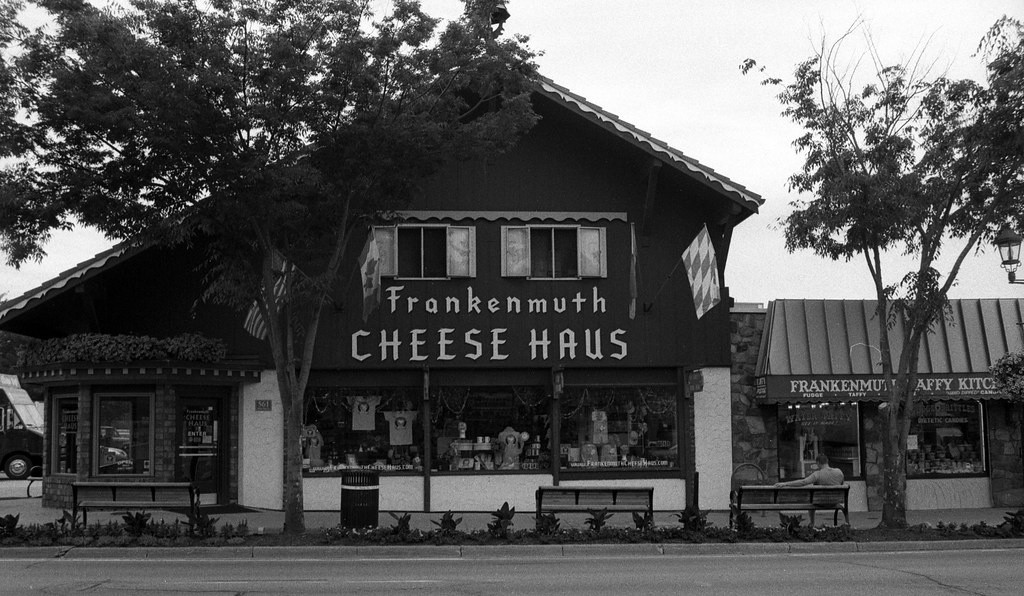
535;486;654;526
70;482;201;533
26;466;43;498
728;483;850;529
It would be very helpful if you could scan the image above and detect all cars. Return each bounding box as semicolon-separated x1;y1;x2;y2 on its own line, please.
100;423;132;467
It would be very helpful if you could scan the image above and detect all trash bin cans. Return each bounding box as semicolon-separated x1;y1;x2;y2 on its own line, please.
341;468;380;530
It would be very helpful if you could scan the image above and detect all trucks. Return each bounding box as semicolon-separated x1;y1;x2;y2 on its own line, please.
0;373;68;480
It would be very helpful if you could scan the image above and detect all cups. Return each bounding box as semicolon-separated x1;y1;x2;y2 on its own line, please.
476;436;490;443
526;435;541;456
475;462;480;470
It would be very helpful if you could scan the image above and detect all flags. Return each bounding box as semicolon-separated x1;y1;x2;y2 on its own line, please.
358;228;383;324
243;252;295;341
629;224;638;319
681;224;722;320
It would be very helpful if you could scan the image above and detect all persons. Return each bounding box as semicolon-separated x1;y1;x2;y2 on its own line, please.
774;453;843;527
498;427;524;471
